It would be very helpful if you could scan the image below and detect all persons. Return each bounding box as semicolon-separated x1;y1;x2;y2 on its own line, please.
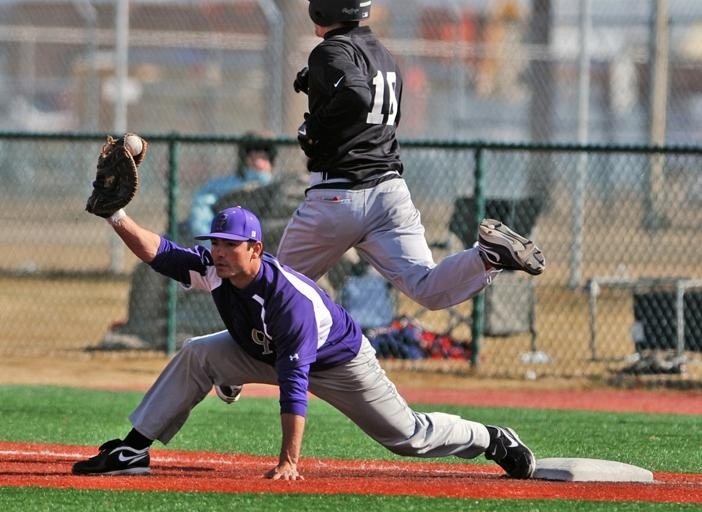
103;133;277;350
70;136;537;479
216;0;546;402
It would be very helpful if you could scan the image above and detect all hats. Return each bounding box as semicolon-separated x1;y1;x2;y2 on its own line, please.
194;206;261;241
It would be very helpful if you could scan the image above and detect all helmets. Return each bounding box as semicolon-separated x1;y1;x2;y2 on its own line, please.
308;0;371;27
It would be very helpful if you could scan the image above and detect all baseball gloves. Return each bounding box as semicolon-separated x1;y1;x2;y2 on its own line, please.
84;132;148;218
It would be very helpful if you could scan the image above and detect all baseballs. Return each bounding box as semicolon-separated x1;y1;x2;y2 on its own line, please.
125;136;142;156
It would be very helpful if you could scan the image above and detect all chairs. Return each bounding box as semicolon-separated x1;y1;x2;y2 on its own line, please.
585;274;702;376
410;196;543;354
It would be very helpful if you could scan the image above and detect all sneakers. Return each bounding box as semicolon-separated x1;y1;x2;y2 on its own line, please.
72;439;150;475
215;384;242;403
478;219;545;275
485;425;535;479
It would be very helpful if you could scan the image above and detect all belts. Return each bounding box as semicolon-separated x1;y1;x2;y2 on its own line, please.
323;172;349;180
